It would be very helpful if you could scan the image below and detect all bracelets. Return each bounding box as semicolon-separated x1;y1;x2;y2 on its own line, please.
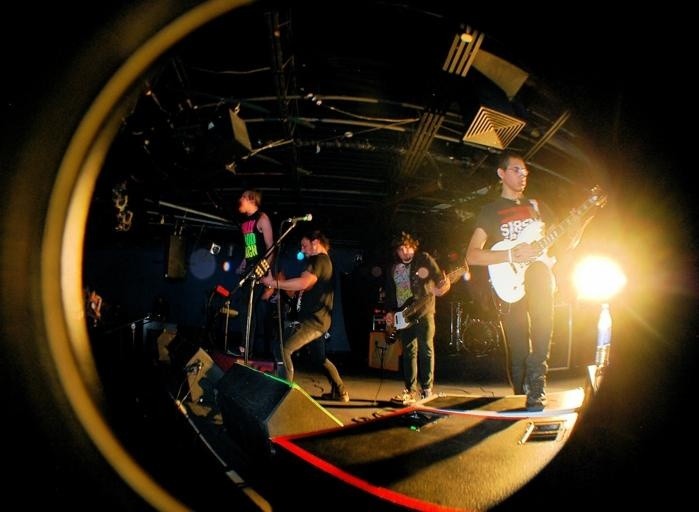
509;250;514;265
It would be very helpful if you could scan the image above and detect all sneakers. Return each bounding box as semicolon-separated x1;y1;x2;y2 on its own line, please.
390;388;420;407
227;345;249;357
320;392;351;403
264;364;288;381
523;375;548;411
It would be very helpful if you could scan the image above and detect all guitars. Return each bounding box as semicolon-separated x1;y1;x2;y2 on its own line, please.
487;185;607;304
384;266;466;345
252;271;297;310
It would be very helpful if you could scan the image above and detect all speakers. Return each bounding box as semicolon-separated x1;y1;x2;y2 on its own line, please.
143;320;584;512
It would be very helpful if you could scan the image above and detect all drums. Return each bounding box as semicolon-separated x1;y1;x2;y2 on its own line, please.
462;319;499;358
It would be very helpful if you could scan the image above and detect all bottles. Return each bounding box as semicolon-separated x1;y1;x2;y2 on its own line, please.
595;304;613;365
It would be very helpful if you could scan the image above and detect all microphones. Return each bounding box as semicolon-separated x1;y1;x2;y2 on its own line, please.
287;212;313;224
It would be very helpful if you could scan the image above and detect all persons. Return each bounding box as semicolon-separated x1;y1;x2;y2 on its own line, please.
236;188;276;357
259;227;352;404
384;222;449;404
464;148;580;413
